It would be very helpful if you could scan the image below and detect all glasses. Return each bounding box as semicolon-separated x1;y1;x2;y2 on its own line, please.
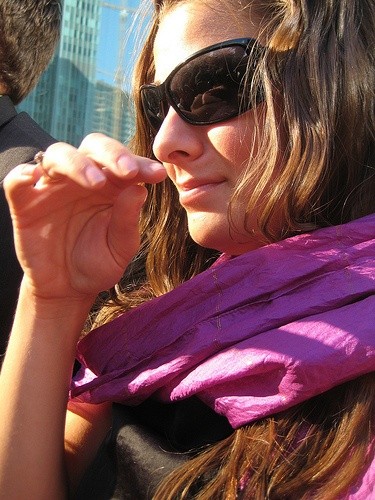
140;38;265;130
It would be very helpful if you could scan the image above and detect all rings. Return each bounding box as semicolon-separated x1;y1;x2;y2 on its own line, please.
34;151;62;182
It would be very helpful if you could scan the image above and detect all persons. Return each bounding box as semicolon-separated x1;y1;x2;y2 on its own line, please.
0;0;375;500
0;0;82;377
